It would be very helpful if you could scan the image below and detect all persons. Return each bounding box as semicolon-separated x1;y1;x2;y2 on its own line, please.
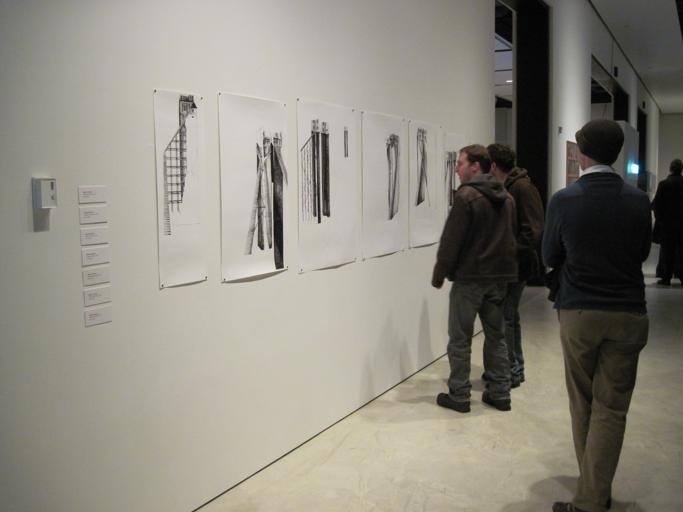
649;159;683;287
485;143;545;389
430;142;521;413
539;119;654;512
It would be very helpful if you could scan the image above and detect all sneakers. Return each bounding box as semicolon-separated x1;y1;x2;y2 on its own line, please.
482;373;524;411
657;280;670;286
438;392;470;412
552;499;611;511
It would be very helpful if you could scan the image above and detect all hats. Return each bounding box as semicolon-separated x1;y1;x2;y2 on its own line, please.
575;119;624;165
